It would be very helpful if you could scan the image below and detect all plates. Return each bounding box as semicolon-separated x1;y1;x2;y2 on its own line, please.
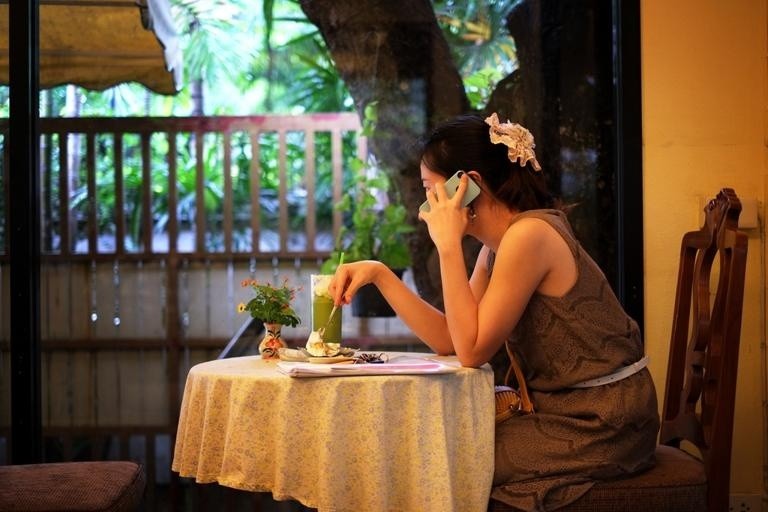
278;347;355;362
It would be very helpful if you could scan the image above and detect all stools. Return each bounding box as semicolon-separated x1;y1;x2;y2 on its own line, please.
0;461;147;512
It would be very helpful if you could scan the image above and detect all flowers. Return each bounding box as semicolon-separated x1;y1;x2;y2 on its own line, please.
235;277;303;329
484;112;545;172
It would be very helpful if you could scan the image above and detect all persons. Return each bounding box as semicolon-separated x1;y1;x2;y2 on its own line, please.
329;113;660;511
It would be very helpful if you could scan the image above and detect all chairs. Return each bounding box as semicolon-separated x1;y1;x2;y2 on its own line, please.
551;187;748;511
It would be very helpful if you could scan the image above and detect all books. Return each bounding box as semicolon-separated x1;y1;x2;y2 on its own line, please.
276;361;458;379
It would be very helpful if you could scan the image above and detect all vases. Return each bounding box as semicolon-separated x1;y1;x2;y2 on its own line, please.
258;323;288;359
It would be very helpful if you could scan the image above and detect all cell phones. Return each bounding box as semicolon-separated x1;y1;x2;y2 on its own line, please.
419;169;482;214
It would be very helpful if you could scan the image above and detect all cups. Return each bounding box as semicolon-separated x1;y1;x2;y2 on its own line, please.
311;274;342;345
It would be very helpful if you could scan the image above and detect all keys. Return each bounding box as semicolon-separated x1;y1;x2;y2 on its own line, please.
357;351;389;364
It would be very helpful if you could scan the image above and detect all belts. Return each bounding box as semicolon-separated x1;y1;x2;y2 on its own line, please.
570;354;651;388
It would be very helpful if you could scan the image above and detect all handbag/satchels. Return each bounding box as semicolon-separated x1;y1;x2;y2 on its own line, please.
494;385;522;426
